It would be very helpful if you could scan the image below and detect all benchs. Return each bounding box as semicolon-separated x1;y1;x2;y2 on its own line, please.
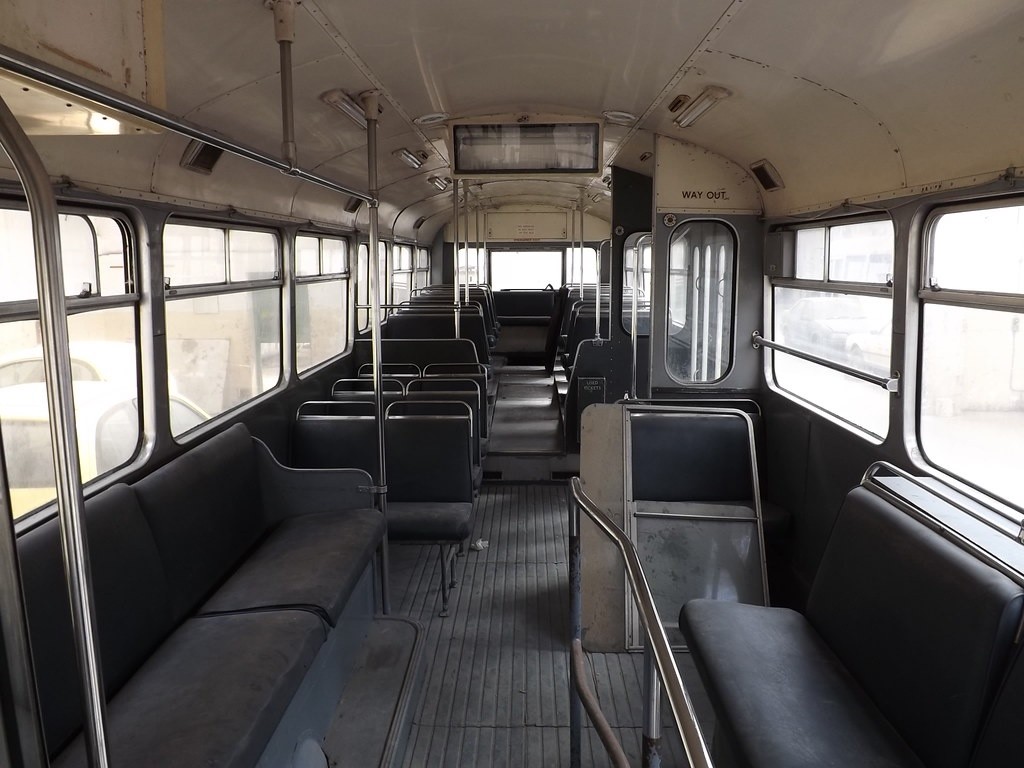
678;460;1024;768
0;423;388;768
290;283;795;617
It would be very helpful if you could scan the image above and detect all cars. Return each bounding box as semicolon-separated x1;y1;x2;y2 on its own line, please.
0;336;209;520
778;292;893;375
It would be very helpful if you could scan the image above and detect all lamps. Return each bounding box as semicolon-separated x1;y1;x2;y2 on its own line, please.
673;86;729;129
448;193;463;203
428;177;447;192
321;88;380;131
391;149;421;169
